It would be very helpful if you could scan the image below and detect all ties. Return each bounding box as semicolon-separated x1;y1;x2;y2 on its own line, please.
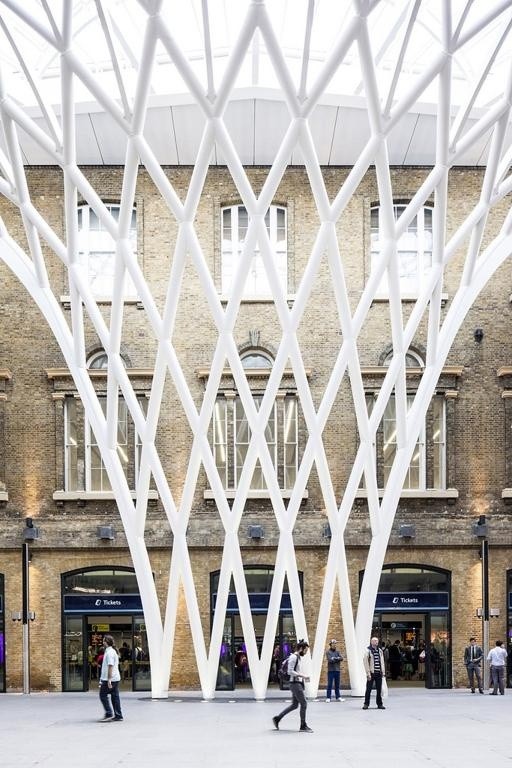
472;647;475;659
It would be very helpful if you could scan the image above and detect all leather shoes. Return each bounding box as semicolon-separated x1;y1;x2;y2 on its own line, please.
471;690;504;694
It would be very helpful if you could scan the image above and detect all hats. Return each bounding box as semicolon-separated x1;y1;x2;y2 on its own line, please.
329;640;337;644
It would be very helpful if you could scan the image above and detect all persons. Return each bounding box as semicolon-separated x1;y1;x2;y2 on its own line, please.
235;646;247;682
271;639;315;734
97;634;124;722
361;636;386;710
487;640;509;695
463;637;485;694
324;639;346;703
379;638;437;683
75;641;146;680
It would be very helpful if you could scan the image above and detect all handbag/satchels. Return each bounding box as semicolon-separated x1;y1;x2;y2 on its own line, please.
277;654;298;690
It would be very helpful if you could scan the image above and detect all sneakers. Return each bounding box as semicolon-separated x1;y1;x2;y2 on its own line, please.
338;697;345;702
100;716;112;722
363;704;368;709
299;725;311;731
378;705;386;708
273;716;280;728
112;717;123;720
326;698;330;702
